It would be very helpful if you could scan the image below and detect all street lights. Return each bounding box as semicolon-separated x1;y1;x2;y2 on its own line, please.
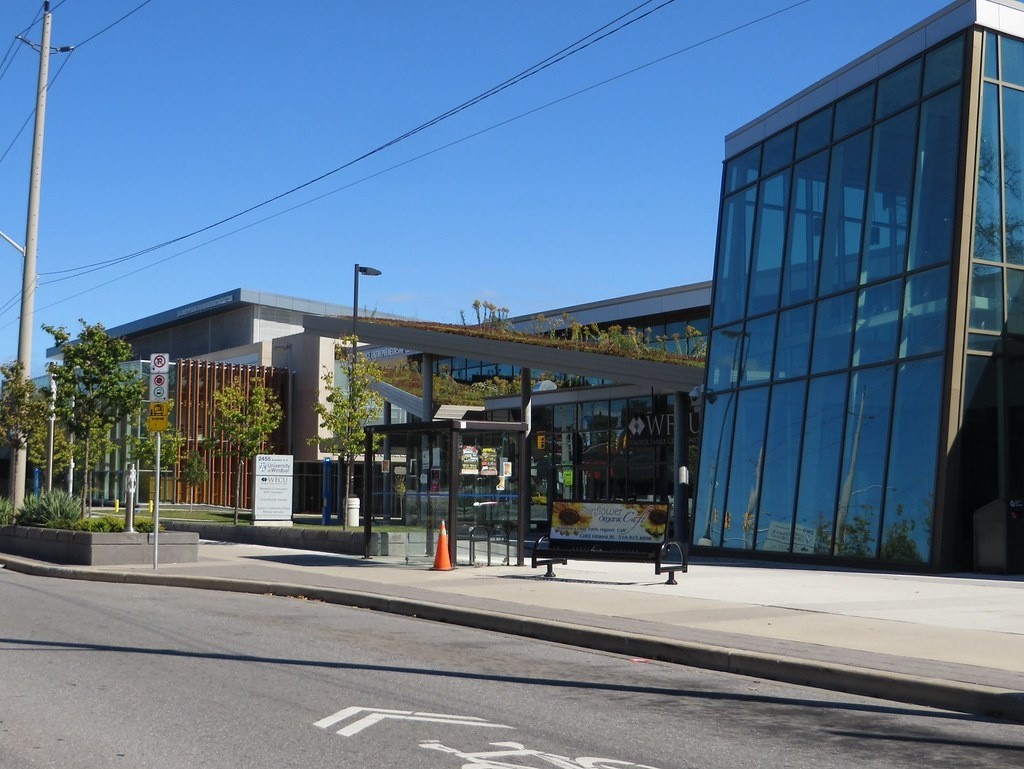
346;263;383;497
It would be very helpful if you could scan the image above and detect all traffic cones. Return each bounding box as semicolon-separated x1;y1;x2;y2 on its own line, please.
429;520;453;571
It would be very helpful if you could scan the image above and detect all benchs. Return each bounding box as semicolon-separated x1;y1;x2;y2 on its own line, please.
531;499;688;585
